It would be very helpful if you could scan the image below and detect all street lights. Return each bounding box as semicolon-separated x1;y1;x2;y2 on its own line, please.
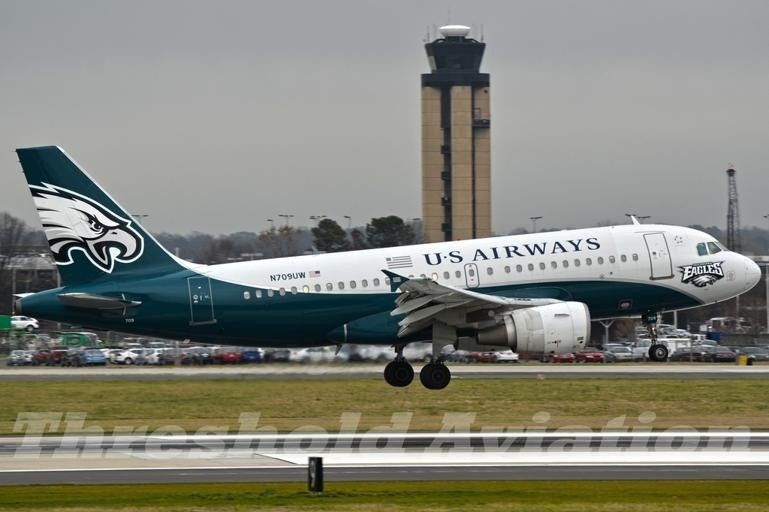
529;214;544;232
267;208;353;235
131;212;149;227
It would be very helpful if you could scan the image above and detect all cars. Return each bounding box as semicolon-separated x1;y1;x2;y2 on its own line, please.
1;313;394;369
404;317;768;367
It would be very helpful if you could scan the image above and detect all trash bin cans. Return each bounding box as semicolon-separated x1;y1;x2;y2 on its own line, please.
737;352;747;366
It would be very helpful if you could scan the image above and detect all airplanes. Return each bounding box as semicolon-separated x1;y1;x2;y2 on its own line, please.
11;143;764;390
